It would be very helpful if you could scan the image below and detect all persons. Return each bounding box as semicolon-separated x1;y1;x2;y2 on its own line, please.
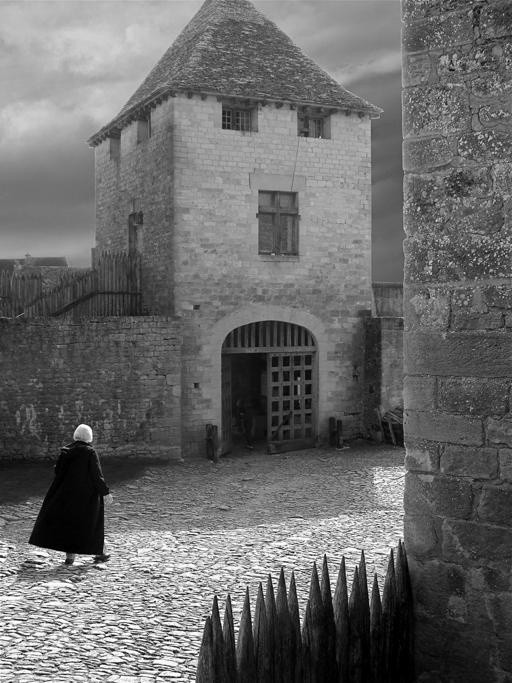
29;423;111;565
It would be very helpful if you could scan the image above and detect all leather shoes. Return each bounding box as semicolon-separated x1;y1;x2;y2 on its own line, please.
95;554;110;560
